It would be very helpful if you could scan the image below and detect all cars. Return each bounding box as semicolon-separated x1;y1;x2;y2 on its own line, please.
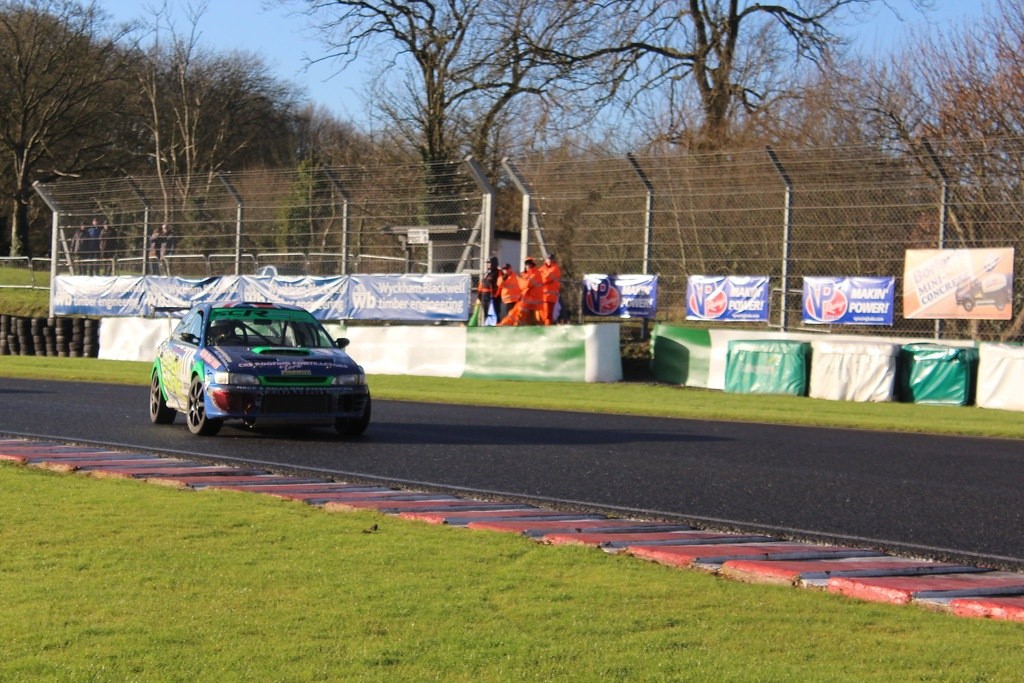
148;302;371;439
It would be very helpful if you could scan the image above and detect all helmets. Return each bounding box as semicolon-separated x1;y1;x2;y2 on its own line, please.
211;319;234;337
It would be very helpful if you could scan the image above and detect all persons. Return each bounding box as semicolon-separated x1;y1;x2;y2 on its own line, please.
99;220;118;275
159;224;177;276
86;217;103;276
476;253;562;327
147;228;162;275
210;318;235;347
70;219;91;275
